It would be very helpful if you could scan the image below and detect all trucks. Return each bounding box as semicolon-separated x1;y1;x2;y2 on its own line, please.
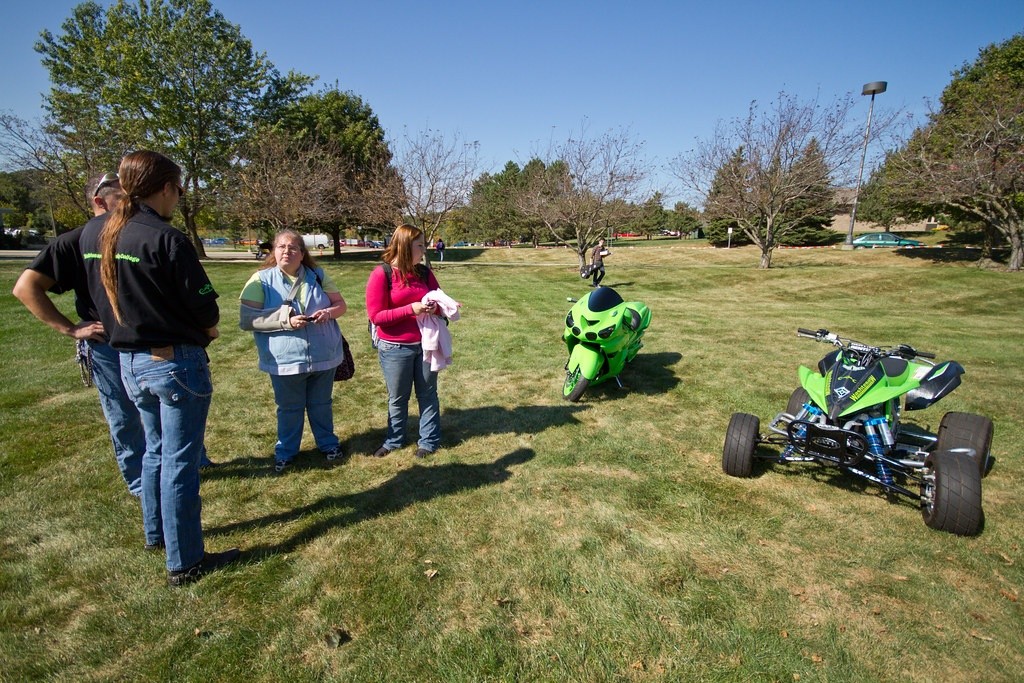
299;234;329;249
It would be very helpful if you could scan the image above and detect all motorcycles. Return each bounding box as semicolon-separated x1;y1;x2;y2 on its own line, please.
562;286;652;403
722;327;994;537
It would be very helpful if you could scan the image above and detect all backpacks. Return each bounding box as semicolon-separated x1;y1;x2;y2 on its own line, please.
368;262;428;349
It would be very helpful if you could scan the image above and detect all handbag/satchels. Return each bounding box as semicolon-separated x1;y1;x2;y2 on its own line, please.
333;336;355;381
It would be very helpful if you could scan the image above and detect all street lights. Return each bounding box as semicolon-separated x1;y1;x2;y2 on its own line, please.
841;82;887;250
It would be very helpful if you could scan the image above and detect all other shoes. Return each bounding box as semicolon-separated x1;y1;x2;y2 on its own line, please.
202;462;223;469
374;446;390;457
593;283;601;288
415;449;431;458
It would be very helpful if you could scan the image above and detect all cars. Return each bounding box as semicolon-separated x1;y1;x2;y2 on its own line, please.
453;240;519;246
330;238;384;249
199;236;264;246
614;231;639;238
852;232;925;249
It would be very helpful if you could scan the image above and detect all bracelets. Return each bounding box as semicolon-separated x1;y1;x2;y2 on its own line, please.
323;309;332;319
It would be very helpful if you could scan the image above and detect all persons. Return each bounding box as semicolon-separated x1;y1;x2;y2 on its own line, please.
436;239;445;261
366;224;449;459
12;150;241;587
590;237;613;289
238;230;347;473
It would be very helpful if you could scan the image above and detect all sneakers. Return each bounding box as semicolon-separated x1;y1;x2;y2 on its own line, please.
327;446;343;462
144;542;165;549
168;549;241;585
275;459;292;471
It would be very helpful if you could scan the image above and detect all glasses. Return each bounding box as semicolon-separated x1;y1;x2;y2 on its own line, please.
94;172;119;196
175;183;186;198
